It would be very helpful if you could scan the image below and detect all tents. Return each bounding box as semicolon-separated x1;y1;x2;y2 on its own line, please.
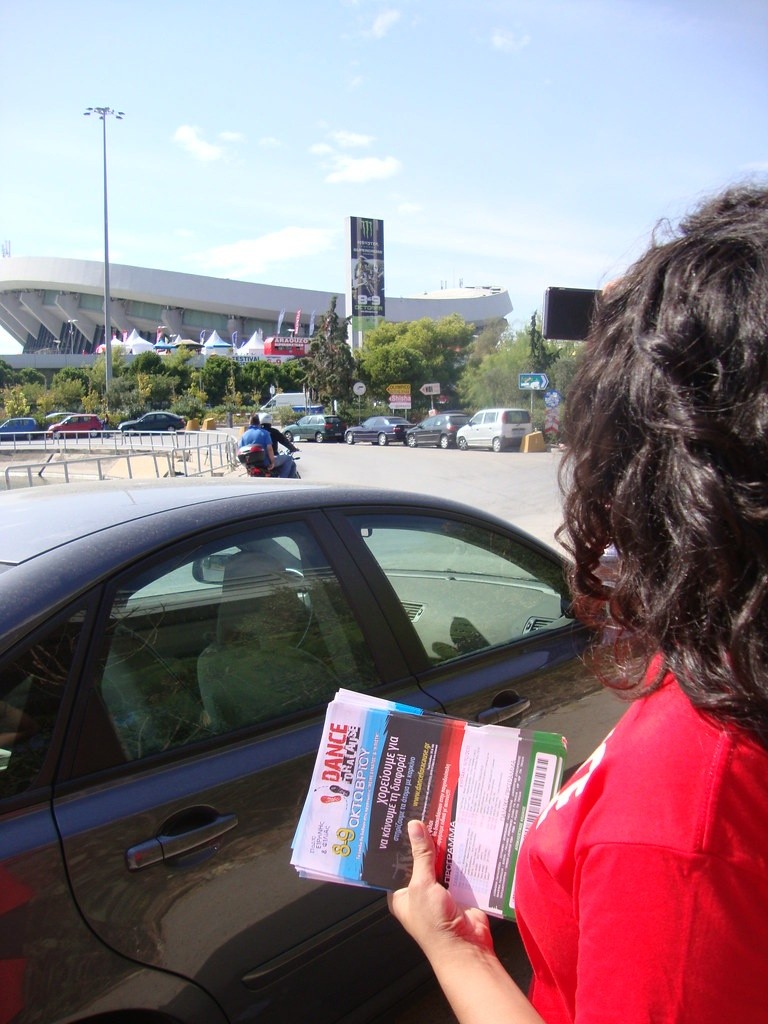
100;329;265;354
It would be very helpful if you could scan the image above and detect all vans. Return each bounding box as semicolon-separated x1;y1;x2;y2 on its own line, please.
456;408;533;452
260;392;311;408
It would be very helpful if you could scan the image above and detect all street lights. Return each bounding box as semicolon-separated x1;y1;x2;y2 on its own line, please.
84;107;125;390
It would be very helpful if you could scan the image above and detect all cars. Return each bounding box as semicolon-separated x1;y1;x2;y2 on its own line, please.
344;415;417;446
282;415;347;443
0;418;40;440
118;412;186;436
45;412;104;438
1;477;635;1024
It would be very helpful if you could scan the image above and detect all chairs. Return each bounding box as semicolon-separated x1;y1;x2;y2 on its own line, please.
197;551;349;739
69;620;210;763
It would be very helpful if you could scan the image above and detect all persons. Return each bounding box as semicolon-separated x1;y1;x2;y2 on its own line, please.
260;414;297;478
238;417;292;478
387;188;767;1024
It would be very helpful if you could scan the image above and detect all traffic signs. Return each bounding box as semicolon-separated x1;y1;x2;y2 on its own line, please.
419;382;440;395
389;402;411;409
518;373;549;390
386;384;411;394
387;394;411;402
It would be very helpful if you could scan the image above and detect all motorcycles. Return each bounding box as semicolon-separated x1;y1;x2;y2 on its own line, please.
237;444;300;477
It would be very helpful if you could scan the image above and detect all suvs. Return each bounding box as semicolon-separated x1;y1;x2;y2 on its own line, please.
406;409;470;448
